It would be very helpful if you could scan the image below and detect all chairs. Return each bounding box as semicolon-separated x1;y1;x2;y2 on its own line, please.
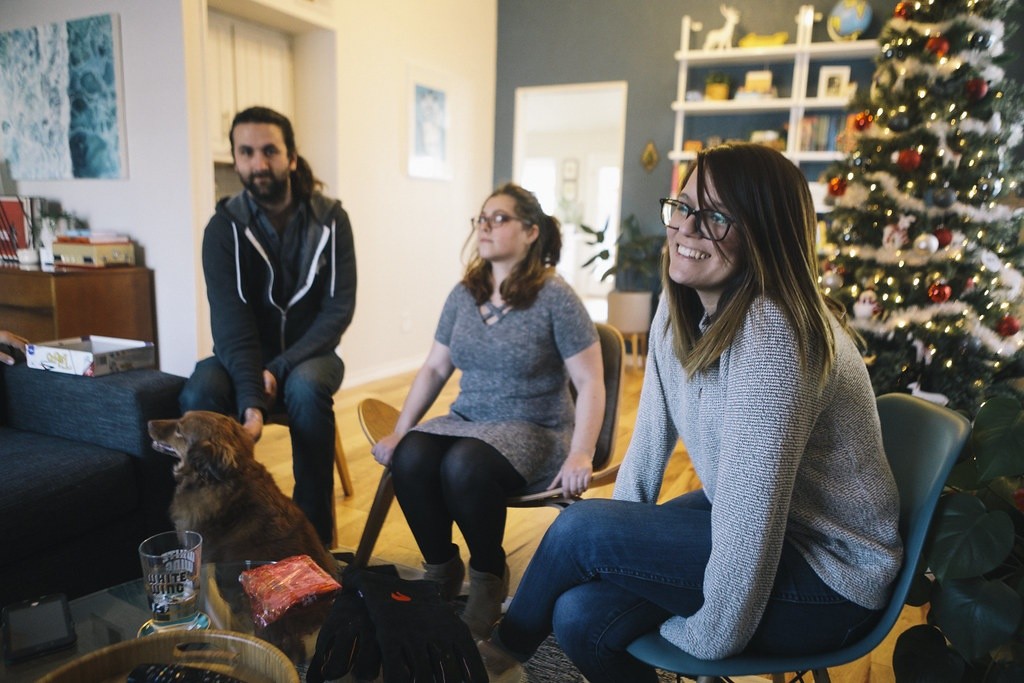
629;394;971;683
355;326;628;564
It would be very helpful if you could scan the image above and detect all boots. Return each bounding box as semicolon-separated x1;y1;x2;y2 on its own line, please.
460;547;509;639
422;544;464;602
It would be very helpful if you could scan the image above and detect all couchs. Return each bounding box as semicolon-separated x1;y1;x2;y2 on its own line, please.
1;342;189;616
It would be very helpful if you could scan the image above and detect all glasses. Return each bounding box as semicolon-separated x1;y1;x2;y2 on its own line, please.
658;197;734;240
471;212;533;232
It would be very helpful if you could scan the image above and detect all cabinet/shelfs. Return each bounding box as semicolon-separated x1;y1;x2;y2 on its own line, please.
666;6;888;215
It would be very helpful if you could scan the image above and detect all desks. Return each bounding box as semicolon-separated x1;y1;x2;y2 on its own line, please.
0;263;158;369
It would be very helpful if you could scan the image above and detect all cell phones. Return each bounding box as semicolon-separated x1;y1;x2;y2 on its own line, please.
0;592;78;665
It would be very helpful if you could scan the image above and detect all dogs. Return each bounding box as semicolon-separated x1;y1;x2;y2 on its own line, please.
147;410;341;635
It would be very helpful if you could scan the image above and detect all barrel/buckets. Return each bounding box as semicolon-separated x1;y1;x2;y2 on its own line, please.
36;630;301;683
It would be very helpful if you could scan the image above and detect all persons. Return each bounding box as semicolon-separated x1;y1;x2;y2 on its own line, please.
181;106;357;552
371;182;606;642
480;144;905;683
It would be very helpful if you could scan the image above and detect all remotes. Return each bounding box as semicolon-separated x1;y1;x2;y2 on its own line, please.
127;663;248;683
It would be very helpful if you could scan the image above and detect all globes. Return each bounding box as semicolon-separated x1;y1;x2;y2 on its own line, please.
827;0;873;41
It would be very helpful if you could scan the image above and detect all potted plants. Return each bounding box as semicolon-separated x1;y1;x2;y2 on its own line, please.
583;219;668;331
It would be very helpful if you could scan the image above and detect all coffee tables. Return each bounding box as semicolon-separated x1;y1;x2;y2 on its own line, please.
0;555;521;683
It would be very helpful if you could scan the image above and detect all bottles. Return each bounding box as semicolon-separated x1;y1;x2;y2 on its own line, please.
817;215;826;245
705;70;729;99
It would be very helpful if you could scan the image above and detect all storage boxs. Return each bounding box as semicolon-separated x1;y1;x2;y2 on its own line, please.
25;333;152;378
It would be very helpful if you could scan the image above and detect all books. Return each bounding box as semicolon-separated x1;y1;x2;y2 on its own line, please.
0;197;49;261
802;113;846;151
57;230;129;244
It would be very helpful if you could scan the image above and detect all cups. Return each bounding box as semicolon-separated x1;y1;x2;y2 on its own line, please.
39;248;55;272
745;70;772;92
139;531;201;632
17;249;39;263
